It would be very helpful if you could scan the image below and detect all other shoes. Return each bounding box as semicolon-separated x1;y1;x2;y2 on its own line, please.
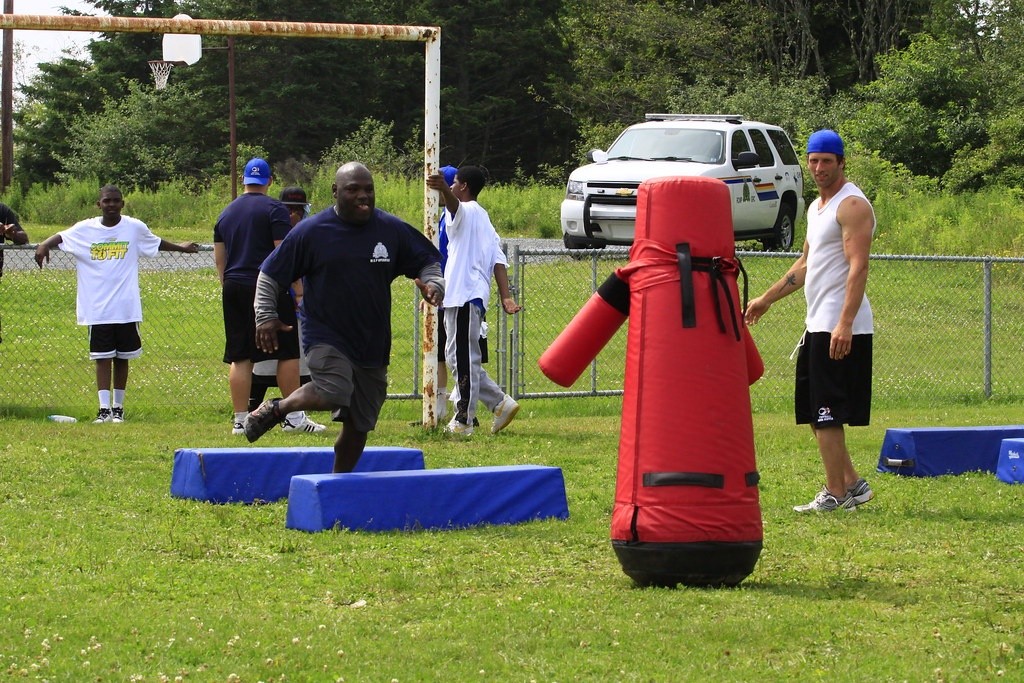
91;405;113;424
112;404;124;423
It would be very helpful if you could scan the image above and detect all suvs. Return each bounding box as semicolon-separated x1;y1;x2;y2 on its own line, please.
560;111;805;261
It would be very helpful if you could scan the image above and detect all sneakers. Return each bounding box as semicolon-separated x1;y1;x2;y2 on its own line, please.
790;485;856;511
244;397;285;443
445;421;476;440
230;419;249;435
282;411;326;434
845;477;876;505
489;394;521;435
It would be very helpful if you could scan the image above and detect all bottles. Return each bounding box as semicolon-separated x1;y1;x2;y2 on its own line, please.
47;415;76;423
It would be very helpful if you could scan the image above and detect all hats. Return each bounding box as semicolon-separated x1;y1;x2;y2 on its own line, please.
278;187;309;208
437;162;461;193
807;129;847;157
242;158;272;189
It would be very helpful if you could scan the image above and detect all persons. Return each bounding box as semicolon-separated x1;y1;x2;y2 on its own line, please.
427;165;521;438
242;162;445;473
213;158;326;433
35;185;200;422
0;202;30;276
741;129;877;512
